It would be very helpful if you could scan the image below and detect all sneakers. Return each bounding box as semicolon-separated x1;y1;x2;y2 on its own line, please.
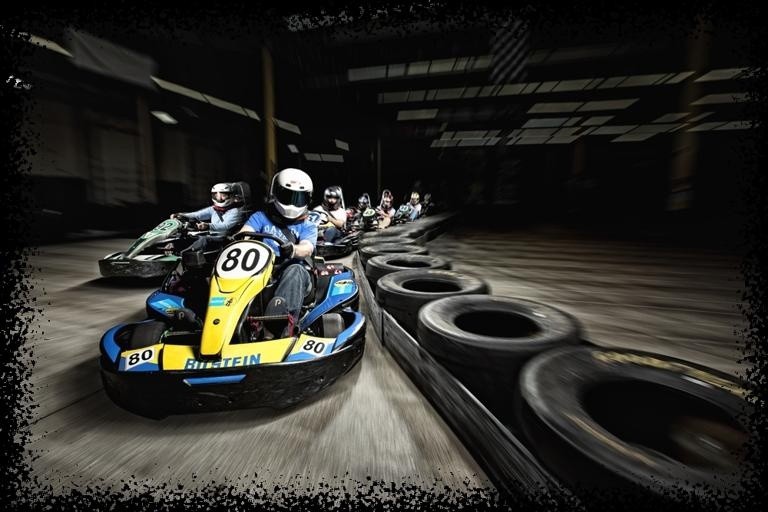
262;296;288;340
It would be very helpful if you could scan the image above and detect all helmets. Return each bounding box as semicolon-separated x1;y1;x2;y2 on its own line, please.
410;192;420;205
270;167;315;219
324;186;342;208
358;196;369;208
210;183;233;207
381;196;393;209
424;193;433;204
